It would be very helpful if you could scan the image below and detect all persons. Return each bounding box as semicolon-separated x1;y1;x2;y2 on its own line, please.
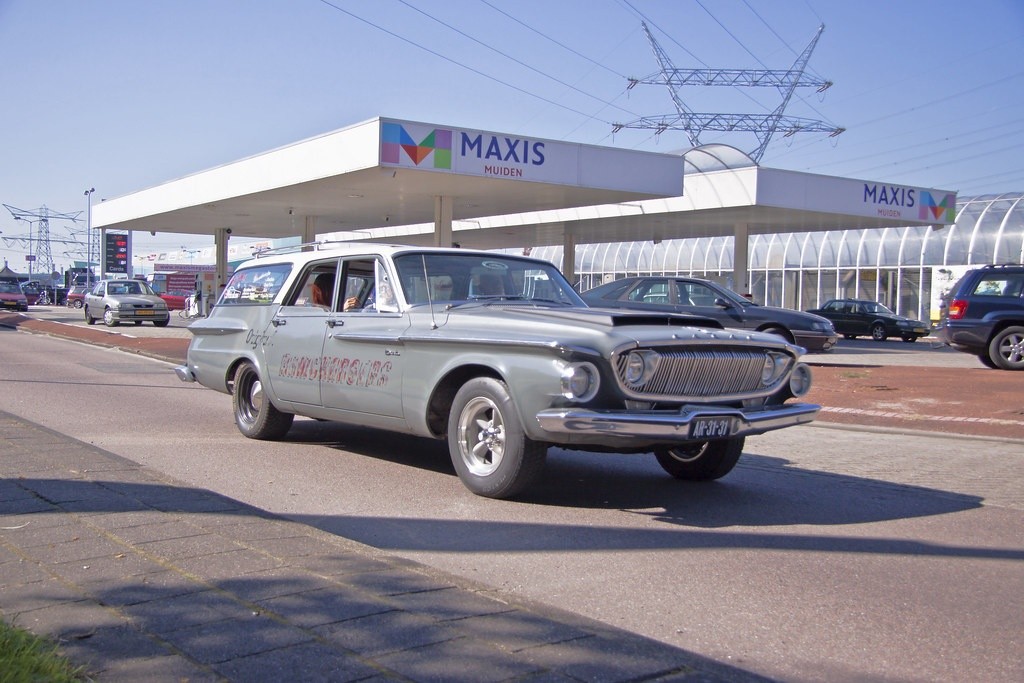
311;273;361;310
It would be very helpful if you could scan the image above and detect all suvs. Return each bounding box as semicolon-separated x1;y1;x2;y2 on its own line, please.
936;263;1024;371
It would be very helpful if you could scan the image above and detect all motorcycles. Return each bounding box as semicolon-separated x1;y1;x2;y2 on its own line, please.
40;291;51;305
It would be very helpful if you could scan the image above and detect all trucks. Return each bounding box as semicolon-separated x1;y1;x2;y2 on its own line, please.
20;281;70;306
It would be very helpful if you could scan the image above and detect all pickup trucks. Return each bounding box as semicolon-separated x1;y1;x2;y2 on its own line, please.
175;247;821;498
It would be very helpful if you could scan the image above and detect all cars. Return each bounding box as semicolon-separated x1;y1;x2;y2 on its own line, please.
583;277;839;353
67;287;87;309
83;279;170;327
806;299;931;343
160;289;194;311
0;281;28;312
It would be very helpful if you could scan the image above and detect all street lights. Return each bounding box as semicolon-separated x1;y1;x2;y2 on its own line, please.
84;188;96;292
